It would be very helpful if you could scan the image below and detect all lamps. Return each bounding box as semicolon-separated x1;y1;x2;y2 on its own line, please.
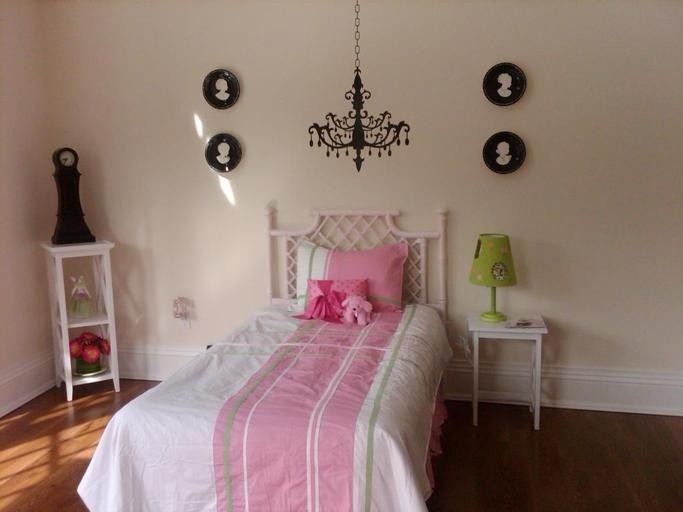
304;1;412;175
466;231;520;323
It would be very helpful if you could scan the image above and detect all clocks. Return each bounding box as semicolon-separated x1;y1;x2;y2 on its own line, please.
50;147;98;245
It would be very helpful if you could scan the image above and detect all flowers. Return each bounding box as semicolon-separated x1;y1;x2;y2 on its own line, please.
68;330;111;365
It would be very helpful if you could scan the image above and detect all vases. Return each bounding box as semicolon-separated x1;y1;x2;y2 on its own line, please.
71;354;108;378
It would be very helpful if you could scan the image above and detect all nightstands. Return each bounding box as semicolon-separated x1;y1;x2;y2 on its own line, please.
463;311;549;431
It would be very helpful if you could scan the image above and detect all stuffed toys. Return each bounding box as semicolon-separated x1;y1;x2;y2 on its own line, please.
341;294;373;326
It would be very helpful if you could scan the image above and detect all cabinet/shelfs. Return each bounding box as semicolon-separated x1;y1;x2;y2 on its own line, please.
37;237;120;402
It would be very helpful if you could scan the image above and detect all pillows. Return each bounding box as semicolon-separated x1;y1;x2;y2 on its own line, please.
293;236;411;314
303;277;371;321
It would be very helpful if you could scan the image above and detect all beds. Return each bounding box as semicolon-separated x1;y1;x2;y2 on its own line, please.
77;203;457;512
